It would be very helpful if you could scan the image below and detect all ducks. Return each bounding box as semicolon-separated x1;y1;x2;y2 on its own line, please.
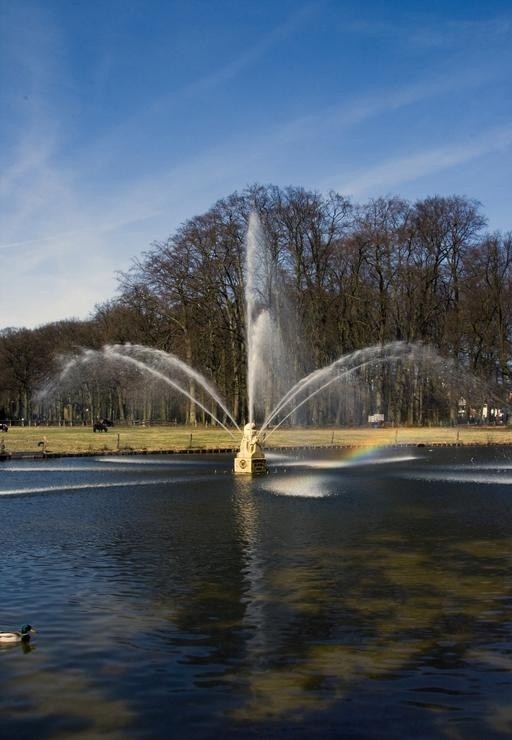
0;624;37;643
